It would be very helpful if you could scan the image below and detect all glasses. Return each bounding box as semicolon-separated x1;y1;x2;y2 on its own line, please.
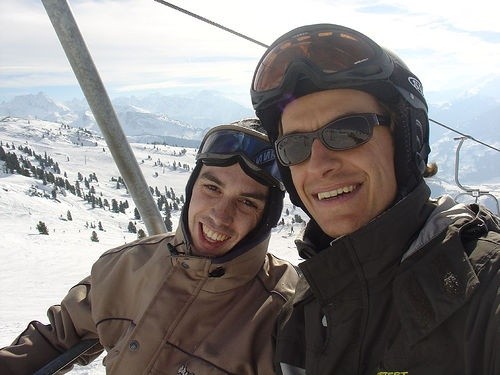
250;22;411;111
194;123;287;191
274;113;389;167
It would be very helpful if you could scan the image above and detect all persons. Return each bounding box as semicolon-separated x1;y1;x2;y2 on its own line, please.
0;116;302;375
270;20;500;375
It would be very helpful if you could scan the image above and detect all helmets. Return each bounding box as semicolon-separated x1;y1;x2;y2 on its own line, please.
262;43;429;206
182;117;286;229
